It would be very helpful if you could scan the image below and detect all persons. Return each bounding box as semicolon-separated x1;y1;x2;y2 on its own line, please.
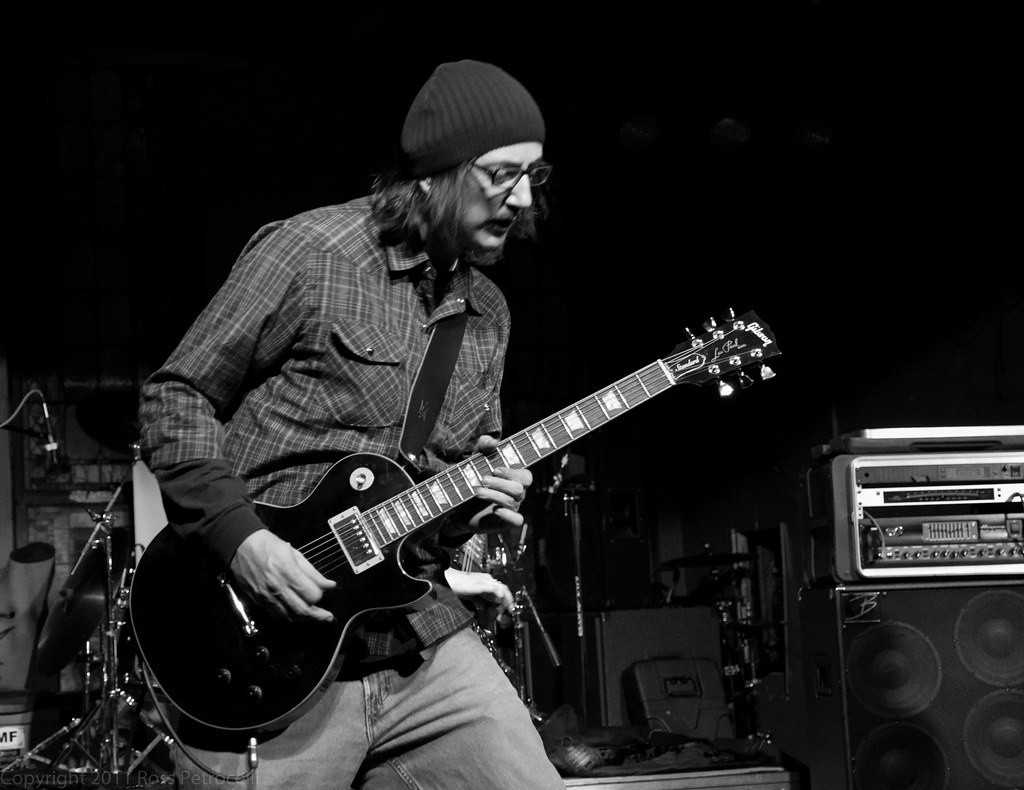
139;62;567;790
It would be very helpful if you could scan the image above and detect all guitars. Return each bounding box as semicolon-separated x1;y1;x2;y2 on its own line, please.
128;305;783;746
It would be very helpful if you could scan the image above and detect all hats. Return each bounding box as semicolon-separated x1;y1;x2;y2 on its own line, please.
401;58;544;178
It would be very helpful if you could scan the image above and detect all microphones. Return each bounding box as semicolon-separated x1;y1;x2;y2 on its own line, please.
38;392;74;497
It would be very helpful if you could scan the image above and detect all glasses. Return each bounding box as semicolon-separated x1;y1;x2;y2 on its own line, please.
469;160;553;187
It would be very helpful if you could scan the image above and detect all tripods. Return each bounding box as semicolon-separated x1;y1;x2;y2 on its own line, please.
41;441;172;789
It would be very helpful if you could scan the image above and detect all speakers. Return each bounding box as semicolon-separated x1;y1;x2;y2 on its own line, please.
796;579;1023;789
